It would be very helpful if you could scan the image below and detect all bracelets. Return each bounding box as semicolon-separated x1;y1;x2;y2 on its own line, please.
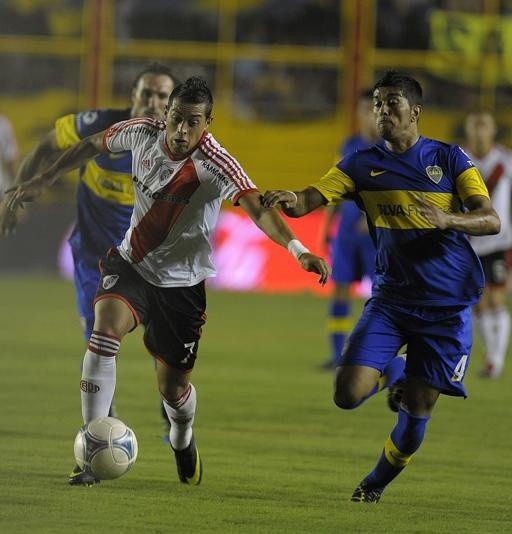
287;239;311;259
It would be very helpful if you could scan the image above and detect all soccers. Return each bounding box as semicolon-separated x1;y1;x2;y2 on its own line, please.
73;417;138;480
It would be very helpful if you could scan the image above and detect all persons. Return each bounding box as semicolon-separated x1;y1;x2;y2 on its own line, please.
444;109;512;381
1;63;180;486
261;73;502;505
310;86;383;374
2;75;334;487
0;109;77;200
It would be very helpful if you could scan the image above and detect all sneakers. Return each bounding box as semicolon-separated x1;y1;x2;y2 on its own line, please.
386;349;409;413
350;481;383;504
167;427;204;487
317;357;506;379
68;464;101;488
